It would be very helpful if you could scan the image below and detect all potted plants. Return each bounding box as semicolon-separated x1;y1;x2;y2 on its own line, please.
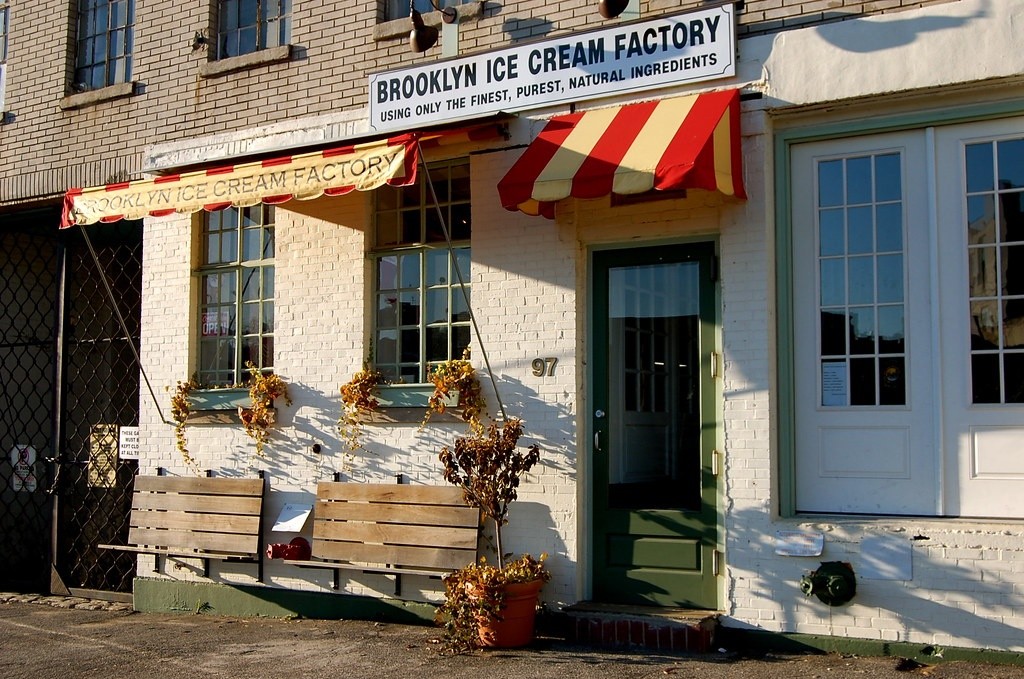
337;360;486;457
165;361;293;475
433;414;552;658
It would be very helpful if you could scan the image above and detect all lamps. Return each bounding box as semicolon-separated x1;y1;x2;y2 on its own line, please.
409;0;457;53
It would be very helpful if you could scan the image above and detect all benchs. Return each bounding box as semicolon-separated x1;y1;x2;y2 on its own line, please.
284;473;479;595
97;467;266;582
